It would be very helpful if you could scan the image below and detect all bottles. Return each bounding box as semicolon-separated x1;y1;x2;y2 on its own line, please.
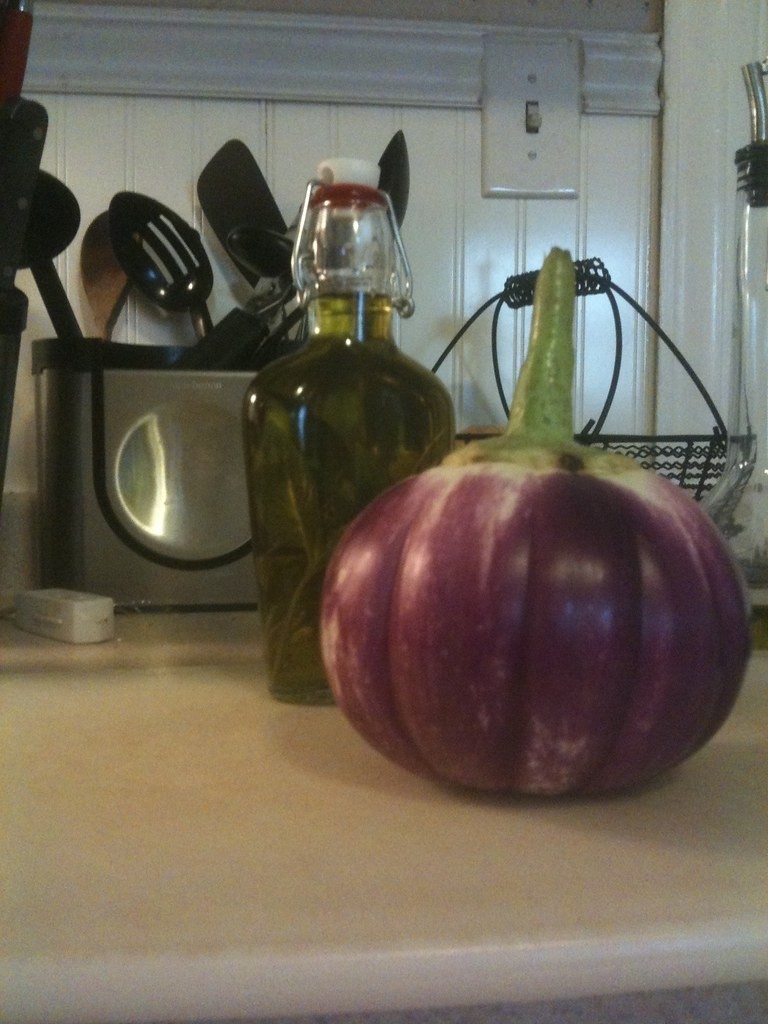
244;157;452;708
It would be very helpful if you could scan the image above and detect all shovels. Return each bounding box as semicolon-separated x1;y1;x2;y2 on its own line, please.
106;190;216;337
198;138;288;290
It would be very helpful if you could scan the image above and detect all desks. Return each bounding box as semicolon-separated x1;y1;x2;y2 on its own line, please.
0;602;768;1024
